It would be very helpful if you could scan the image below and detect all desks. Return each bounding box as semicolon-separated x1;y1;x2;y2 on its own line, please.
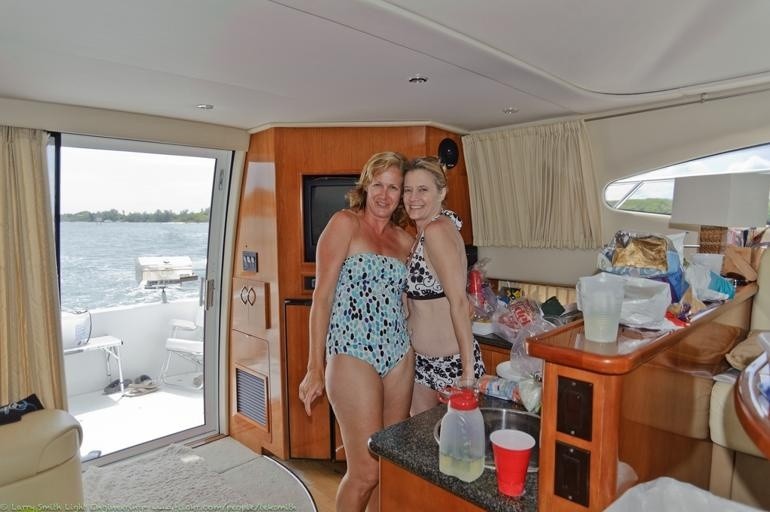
735;352;770;459
63;336;124;394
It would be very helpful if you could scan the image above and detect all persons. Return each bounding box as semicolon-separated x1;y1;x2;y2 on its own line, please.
403;157;486;417
299;151;416;512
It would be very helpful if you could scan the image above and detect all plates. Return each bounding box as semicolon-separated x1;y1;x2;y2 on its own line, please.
496;358;531;382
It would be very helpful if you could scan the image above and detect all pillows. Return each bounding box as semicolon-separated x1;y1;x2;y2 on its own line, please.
725;330;770;371
668;321;741;365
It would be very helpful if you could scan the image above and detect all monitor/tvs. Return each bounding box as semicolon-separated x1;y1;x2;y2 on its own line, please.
301;174;361;262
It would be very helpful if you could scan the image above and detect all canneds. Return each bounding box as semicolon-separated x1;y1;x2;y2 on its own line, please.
469;270;484;314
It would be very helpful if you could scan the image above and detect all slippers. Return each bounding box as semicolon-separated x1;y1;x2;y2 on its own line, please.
104;375;161;397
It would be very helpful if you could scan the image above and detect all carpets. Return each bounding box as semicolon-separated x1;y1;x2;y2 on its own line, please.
83;444;248;512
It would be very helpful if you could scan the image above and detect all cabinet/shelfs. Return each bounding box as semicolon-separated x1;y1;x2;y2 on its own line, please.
287;301;347;460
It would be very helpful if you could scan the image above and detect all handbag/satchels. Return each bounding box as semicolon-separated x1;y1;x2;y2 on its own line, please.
595;231;704;324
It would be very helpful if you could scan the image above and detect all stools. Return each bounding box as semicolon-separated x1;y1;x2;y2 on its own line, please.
0;409;83;512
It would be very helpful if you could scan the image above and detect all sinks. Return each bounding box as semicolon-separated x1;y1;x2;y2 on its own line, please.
433;409;541;472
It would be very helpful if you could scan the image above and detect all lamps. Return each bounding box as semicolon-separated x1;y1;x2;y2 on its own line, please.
670;173;770;254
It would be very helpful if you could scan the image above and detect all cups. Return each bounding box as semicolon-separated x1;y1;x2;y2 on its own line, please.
468;270;484;300
488;429;535;497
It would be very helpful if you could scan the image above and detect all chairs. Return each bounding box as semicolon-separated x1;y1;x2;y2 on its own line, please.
602;477;763;512
623;300;751;492
161;310;204;390
709;247;770;512
